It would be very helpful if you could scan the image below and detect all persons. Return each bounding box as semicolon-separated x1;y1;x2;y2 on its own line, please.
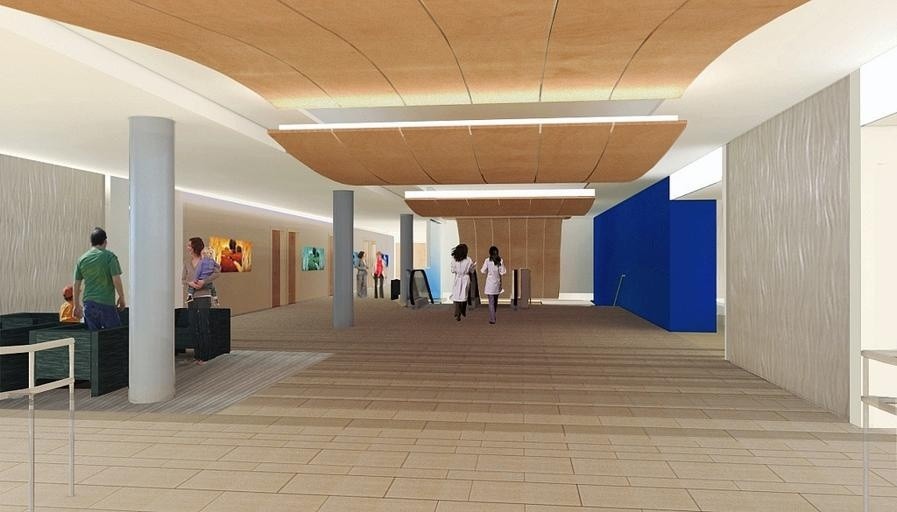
355;251;369;300
58;285;82;322
370;250;386;301
185;246;220;306
311;248;320;265
307;253;321;271
219;240;244;272
181;236;222;367
449;243;475;321
71;226;126;329
229;245;243;266
480;246;507;326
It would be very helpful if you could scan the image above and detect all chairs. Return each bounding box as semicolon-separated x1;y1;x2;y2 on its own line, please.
0;308;229;397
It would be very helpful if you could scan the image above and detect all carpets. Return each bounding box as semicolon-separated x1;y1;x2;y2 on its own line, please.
0;349;333;421
